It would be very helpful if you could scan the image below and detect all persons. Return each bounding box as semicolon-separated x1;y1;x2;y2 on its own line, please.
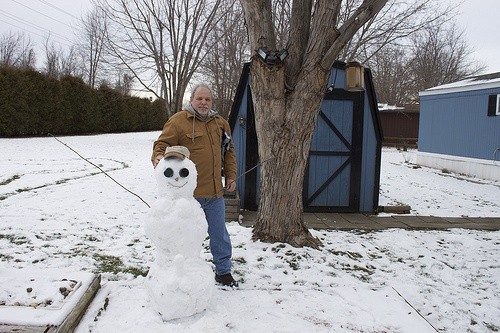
150;85;239;286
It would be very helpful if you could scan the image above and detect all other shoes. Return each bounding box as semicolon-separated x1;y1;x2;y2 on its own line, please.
215;273;238;287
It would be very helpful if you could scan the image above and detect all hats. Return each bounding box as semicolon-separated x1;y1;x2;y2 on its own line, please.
164;146;190;160
